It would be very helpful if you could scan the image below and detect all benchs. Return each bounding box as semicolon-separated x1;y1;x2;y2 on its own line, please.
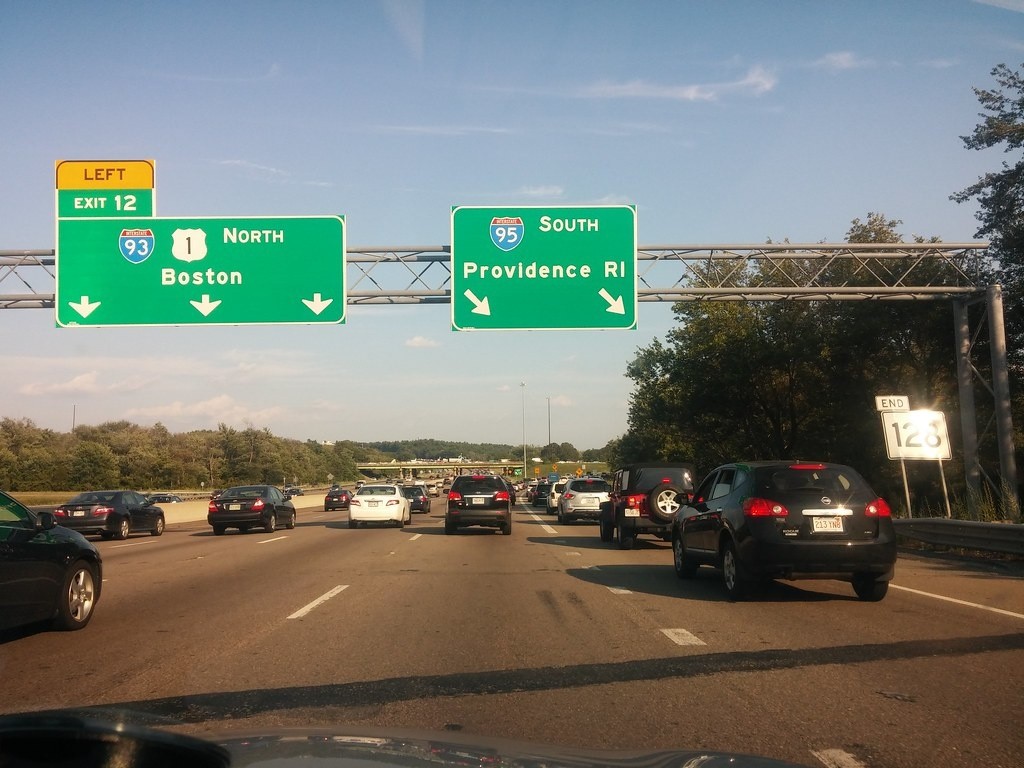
363;491;392;495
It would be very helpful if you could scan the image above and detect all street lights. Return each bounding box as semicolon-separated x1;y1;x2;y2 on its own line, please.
545;396;550;445
519;381;527;477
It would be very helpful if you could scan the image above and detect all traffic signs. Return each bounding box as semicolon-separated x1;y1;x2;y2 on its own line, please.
449;203;639;331
56;158;349;328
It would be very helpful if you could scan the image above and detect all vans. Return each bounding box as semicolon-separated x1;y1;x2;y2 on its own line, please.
531;458;543;462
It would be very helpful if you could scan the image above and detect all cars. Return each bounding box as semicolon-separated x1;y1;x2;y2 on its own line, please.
506;482;516;506
526;483;537;502
368;462;376;465
282;484;303;497
324;490;354;513
206;486;297;533
436;482;444;488
55;492;167;538
386;475;412;484
512;483;519;492
210;489;224;499
0;491;103;636
349;485;412;528
443;479;452;485
329;485;343;491
516;481;524;489
400;485;432;514
523;472;608;486
146;493;182;506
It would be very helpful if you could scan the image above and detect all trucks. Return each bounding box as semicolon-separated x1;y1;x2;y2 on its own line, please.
449;458;466;463
355;480;366;489
501;459;511;463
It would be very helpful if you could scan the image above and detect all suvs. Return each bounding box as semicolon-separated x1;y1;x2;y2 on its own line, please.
599;467;695;546
445;475;515;535
545;481;568;514
531;484;552;506
557;477;613;522
426;484;440;498
671;459;900;602
